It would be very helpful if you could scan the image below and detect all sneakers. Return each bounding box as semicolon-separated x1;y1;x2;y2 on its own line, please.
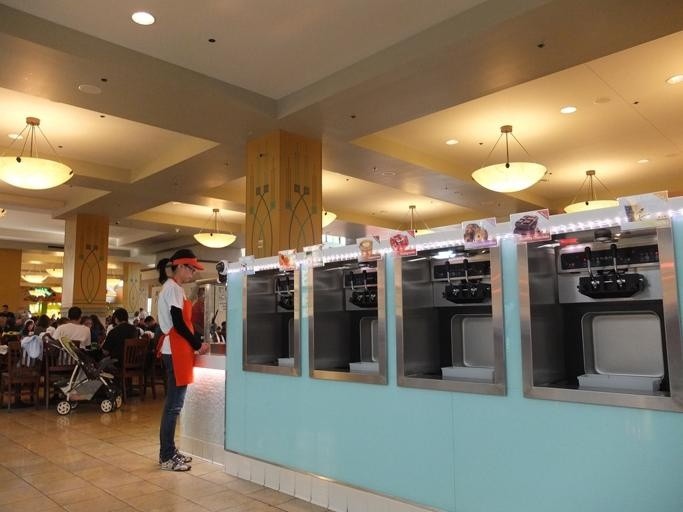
158;451;192;461
161;458;191;471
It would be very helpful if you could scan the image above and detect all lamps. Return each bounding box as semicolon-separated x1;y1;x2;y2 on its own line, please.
395;205;435;237
193;208;236;249
0;117;73;191
322;210;337;229
564;169;620;214
472;123;547;193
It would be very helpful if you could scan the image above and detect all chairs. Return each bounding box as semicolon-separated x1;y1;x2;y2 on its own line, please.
42;333;81;409
147;351;168;399
115;337;147;403
0;340;40;410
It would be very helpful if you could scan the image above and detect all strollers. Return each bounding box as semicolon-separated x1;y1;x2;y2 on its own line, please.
42;333;122;415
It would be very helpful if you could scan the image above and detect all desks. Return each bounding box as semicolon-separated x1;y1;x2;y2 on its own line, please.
2;331;21;346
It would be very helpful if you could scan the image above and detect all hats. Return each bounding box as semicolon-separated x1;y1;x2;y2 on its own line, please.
172;255;204;271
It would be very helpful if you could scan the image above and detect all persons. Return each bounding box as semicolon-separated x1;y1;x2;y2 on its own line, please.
143;316;162;349
20;319;37;337
105;314;115;337
154;248;210;471
108;308;139;372
1;304;16;328
82;315;93;329
133;311;141;323
138;306;146;319
90;314;106;344
34;314;52;334
53;307;92;366
132;319;145;332
0;314;7;332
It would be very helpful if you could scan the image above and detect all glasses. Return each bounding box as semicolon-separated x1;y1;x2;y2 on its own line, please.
185;264;196;273
